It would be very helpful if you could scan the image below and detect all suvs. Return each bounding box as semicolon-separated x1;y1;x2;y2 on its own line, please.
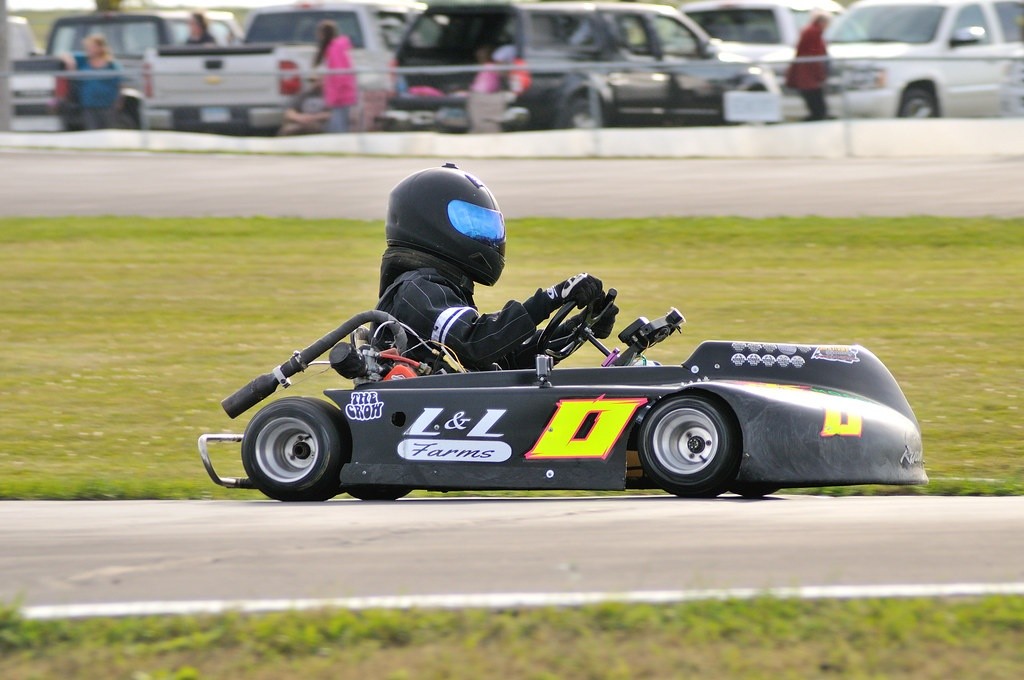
374;1;783;133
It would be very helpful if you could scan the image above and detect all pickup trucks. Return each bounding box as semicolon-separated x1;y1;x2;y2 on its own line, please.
134;1;451;136
8;11;248;134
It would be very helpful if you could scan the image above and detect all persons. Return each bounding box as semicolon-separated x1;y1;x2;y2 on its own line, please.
787;11;834;121
366;165;616;371
181;12;218;47
309;19;358;133
59;35;124;130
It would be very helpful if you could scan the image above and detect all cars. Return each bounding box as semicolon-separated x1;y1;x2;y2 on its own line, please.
817;1;1024;117
674;0;875;115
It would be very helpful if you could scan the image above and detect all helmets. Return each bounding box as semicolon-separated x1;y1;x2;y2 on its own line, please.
386;164;507;286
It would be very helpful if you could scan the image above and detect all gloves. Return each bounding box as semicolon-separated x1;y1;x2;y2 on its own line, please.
566;291;619;339
551;273;603;311
